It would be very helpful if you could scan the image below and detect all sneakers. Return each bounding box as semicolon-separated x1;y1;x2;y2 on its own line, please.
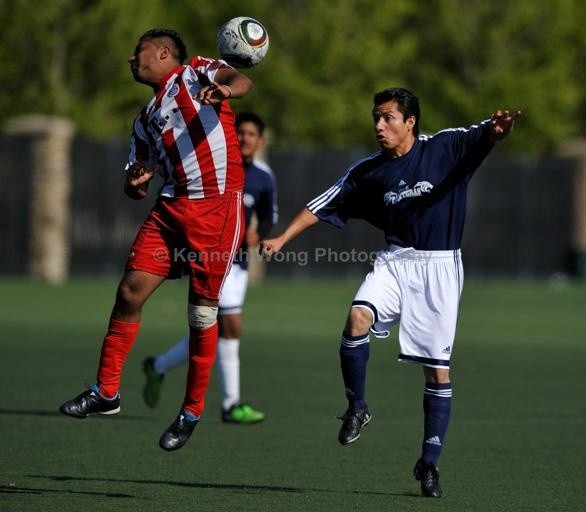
220;400;265;424
338;406;371;446
159;408;201;451
412;459;443;497
59;383;121;418
142;356;164;409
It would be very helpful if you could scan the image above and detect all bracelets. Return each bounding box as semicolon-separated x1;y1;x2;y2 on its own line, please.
220;82;233;99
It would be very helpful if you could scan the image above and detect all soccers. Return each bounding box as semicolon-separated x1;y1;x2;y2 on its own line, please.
217;16;269;68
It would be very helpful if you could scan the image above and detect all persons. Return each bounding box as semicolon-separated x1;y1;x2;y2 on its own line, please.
142;109;280;426
259;84;525;498
59;24;254;451
